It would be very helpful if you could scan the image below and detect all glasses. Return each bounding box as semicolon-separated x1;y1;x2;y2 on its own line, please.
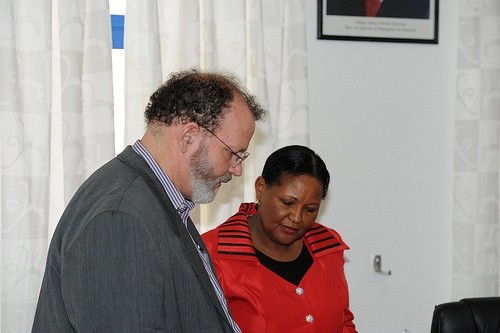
188;113;250;168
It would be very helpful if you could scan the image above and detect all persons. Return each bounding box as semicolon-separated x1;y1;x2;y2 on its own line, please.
199;146;360;333
31;72;264;333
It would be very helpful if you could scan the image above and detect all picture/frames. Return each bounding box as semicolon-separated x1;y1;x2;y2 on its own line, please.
317;0;438;45
